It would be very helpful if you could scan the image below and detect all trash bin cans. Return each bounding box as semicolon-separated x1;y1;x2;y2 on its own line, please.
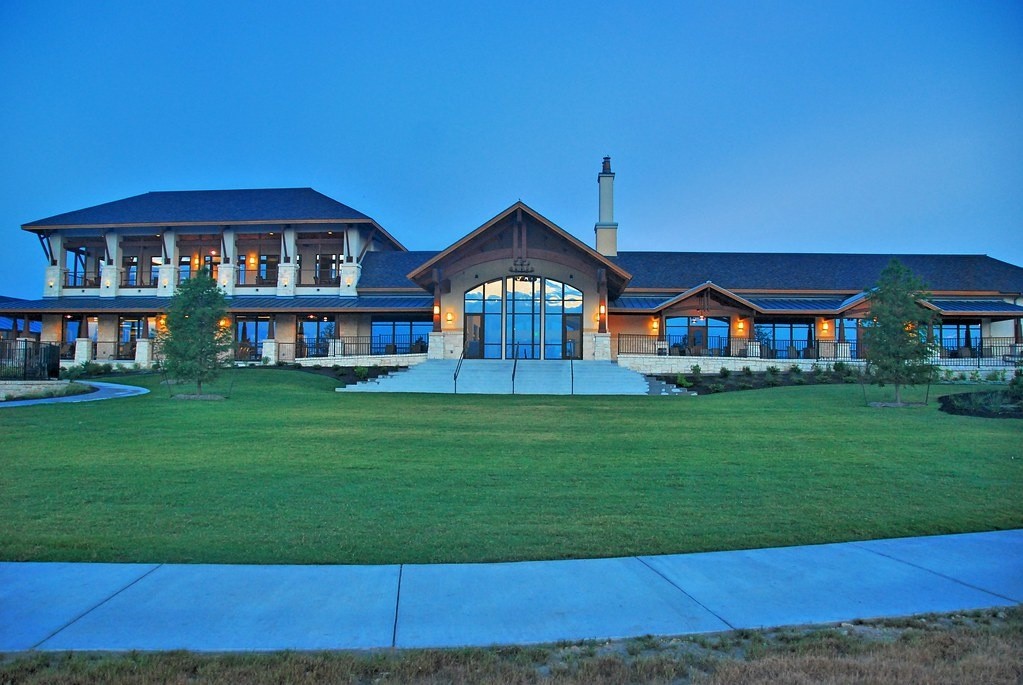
385;345;397;353
566;339;575;358
658;348;667;356
467;341;479;356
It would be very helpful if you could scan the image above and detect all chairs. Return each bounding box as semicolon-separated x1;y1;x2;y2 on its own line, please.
313;276;328;285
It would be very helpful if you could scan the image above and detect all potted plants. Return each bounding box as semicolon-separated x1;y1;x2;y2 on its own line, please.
411;337;428;353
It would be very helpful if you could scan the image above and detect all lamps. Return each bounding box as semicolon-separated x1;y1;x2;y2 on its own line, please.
699;298;704;320
